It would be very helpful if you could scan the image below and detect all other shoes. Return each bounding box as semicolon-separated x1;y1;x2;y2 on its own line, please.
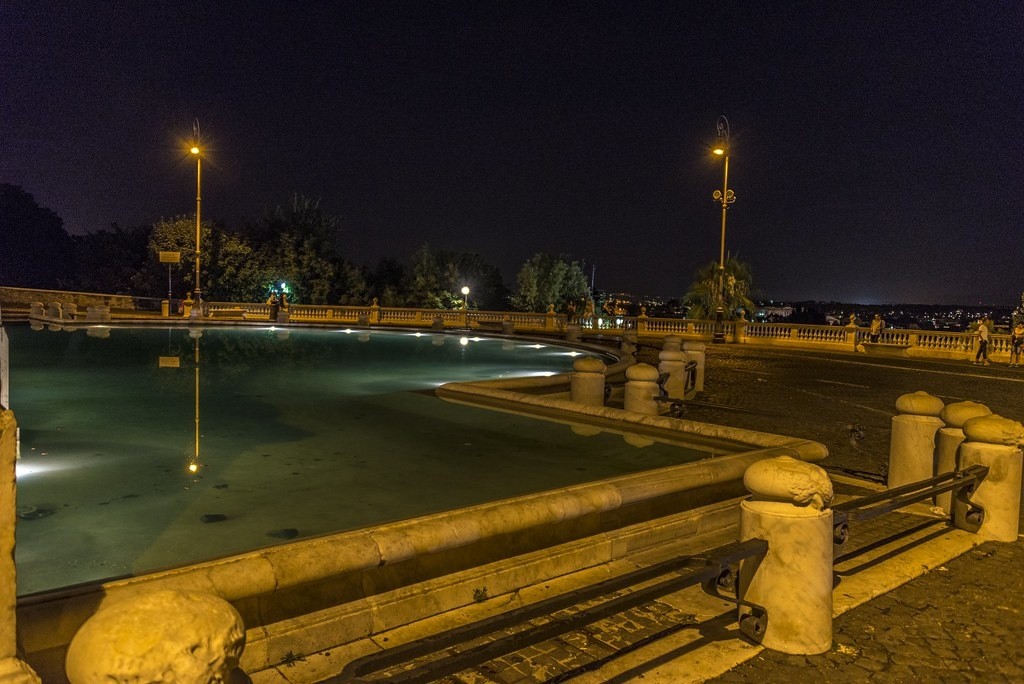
984;363;990;366
1015;363;1020;367
974;362;983;365
1008;362;1014;368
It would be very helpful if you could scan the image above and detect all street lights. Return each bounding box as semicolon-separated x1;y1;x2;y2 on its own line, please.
189;337;202;475
710;113;736;345
460;285;471;324
185;115;206;321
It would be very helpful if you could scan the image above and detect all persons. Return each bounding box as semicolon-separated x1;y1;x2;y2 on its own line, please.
1008;321;1024;368
974;317;991;366
871;314;885;343
567;300;616;329
178;298;185;313
267;291;281;319
